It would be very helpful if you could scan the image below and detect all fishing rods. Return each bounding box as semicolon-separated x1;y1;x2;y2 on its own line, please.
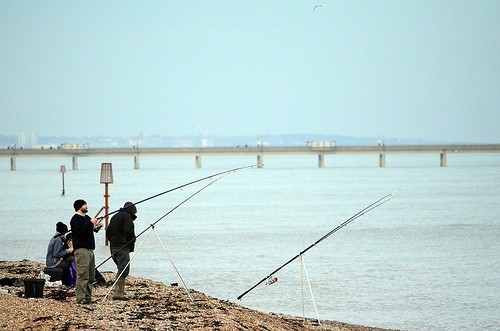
237;189;401;299
53;165;260;238
55;171;231;300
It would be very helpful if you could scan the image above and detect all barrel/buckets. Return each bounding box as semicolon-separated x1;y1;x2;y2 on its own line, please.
22;279;46;298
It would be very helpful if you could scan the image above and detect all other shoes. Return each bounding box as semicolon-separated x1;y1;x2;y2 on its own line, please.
77;299;97;305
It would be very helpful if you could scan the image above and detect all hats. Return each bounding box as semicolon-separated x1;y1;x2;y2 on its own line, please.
124;202;136;214
73;199;87;212
66;233;72;242
56;222;68;234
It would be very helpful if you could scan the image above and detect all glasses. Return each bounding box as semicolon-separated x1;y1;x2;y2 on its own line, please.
69;238;72;241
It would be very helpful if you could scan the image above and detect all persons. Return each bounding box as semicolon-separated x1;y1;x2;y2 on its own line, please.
46;222;112;286
106;202;137;299
70;199;98;305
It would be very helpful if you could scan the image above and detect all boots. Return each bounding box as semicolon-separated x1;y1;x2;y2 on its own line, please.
113;281;129;301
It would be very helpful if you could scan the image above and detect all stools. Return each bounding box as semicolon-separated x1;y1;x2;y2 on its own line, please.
41;267;76;299
